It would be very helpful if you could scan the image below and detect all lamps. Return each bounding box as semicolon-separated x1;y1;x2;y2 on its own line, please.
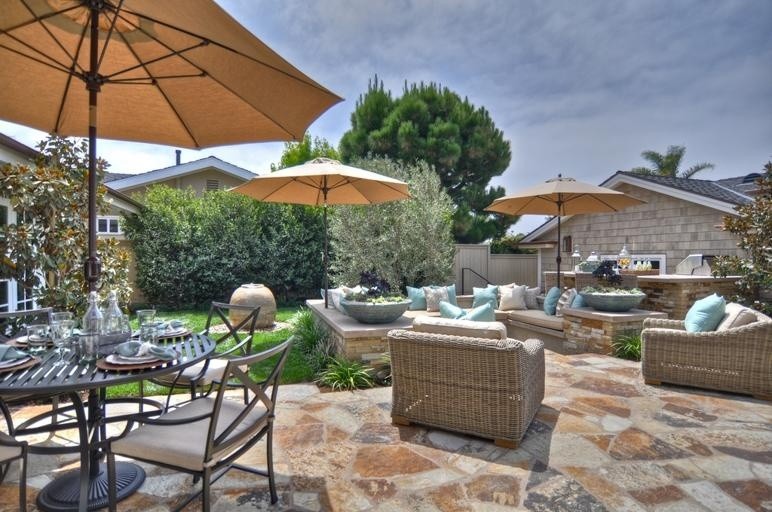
571;244;585;264
620;244;634;257
587;248;602;262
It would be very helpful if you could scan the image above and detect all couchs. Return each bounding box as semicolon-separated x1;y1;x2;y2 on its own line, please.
639;290;772;404
387;313;547;448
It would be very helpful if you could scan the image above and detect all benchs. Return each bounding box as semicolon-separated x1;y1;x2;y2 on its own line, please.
502;288;571;355
399;290;502;337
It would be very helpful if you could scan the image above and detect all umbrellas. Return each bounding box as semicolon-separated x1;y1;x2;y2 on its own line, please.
484;173;647;291
226;156;416;310
1;1;348;512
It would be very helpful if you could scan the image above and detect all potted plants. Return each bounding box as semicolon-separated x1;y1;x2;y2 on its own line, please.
579;260;646;311
337;270;412;323
319;263;335;307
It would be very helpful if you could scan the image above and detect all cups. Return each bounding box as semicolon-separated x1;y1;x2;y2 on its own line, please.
24;309;161;367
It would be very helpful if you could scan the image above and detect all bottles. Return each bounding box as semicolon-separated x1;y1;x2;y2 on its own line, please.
105;290;122;353
617;256;652;273
83;291;104;359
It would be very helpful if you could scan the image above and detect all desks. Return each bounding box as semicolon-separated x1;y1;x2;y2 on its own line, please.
0;316;218;512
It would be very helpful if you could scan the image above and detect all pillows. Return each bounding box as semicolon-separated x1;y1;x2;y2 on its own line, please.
684;293;726;333
405;282;587;322
716;302;758;331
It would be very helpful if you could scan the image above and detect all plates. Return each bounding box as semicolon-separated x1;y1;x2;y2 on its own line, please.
0;322;193;374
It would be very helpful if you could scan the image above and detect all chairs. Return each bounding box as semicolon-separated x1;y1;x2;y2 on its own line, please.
134;298;260;426
0;303;70;445
103;329;297;512
0;412;28;512
